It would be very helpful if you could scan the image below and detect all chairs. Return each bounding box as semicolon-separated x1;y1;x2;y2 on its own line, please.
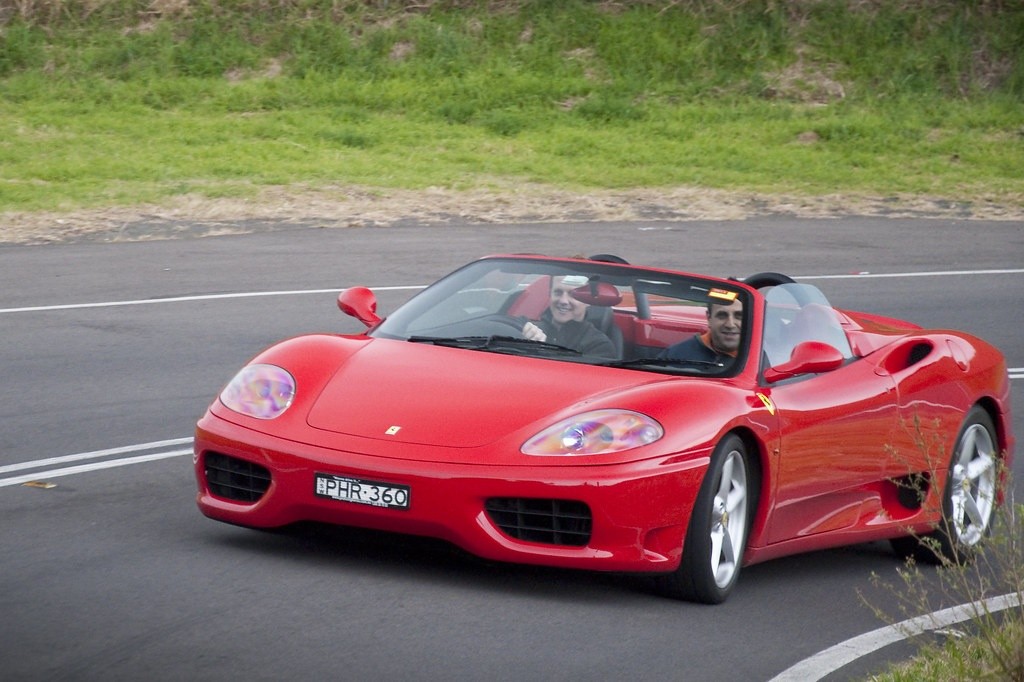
586;305;624;360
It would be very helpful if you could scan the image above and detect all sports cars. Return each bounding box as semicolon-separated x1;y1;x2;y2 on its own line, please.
194;251;1014;605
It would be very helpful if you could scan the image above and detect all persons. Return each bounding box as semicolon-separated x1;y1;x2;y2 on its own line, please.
516;276;615;359
656;298;776;387
